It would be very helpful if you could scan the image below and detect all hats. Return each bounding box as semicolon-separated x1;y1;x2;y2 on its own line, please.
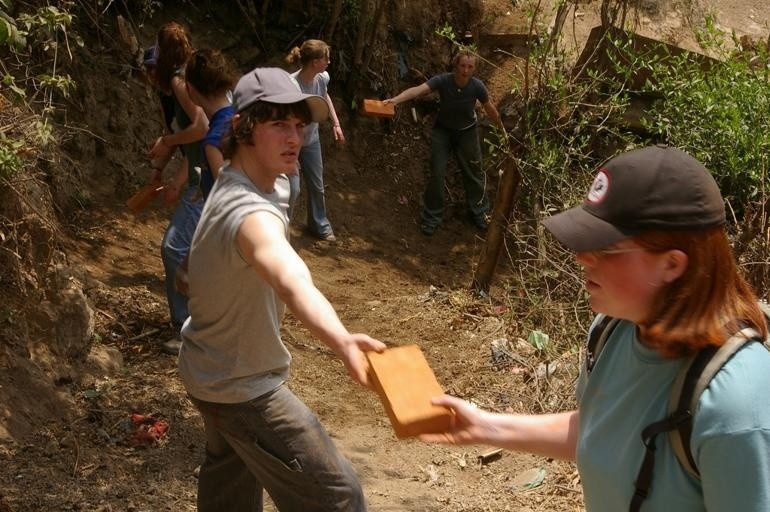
233;67;330;122
540;143;726;251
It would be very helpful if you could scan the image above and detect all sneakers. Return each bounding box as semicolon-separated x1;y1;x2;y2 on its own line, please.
325;234;337;243
420;223;435;235
163;333;183;353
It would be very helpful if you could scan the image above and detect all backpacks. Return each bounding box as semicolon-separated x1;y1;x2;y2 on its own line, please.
586;300;770;512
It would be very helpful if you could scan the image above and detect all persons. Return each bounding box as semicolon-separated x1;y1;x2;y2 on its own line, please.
417;144;770;512
383;51;508;235
